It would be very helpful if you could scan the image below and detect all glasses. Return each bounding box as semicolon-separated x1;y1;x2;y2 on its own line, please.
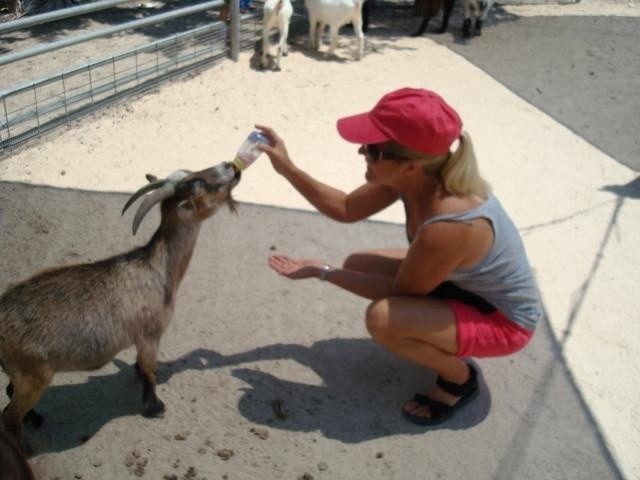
366;145;410;162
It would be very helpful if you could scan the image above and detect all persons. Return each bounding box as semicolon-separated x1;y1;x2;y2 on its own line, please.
254;86;543;427
223;0;258;46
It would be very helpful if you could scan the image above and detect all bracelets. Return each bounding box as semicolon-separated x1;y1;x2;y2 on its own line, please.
320;265;330;281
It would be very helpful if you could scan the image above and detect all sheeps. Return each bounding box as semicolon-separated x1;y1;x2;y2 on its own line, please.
303;0;366;61
257;0;295;71
0;160;243;457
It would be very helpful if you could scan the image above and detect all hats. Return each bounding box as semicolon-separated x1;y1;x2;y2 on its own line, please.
336;87;462;156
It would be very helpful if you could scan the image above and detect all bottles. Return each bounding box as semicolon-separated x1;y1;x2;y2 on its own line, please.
232;129;273;175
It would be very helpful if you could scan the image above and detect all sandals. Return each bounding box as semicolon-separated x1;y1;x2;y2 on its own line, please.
400;363;478;426
